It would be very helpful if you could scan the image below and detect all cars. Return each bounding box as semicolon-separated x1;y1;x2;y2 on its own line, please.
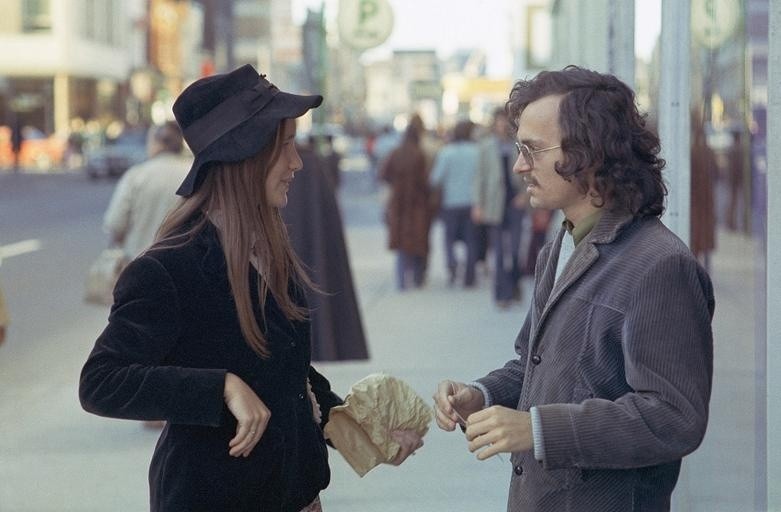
84;123;157;180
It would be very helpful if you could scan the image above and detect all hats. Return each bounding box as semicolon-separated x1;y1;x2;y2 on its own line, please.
166;61;325;197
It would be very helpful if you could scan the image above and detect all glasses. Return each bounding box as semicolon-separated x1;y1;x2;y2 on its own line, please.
509;138;570;171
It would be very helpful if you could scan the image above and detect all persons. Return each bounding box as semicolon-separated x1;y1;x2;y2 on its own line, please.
101;122;195;265
430;60;720;512
318;132;340;190
689;128;720;271
369;105;553;310
75;59;434;511
721;125;755;237
